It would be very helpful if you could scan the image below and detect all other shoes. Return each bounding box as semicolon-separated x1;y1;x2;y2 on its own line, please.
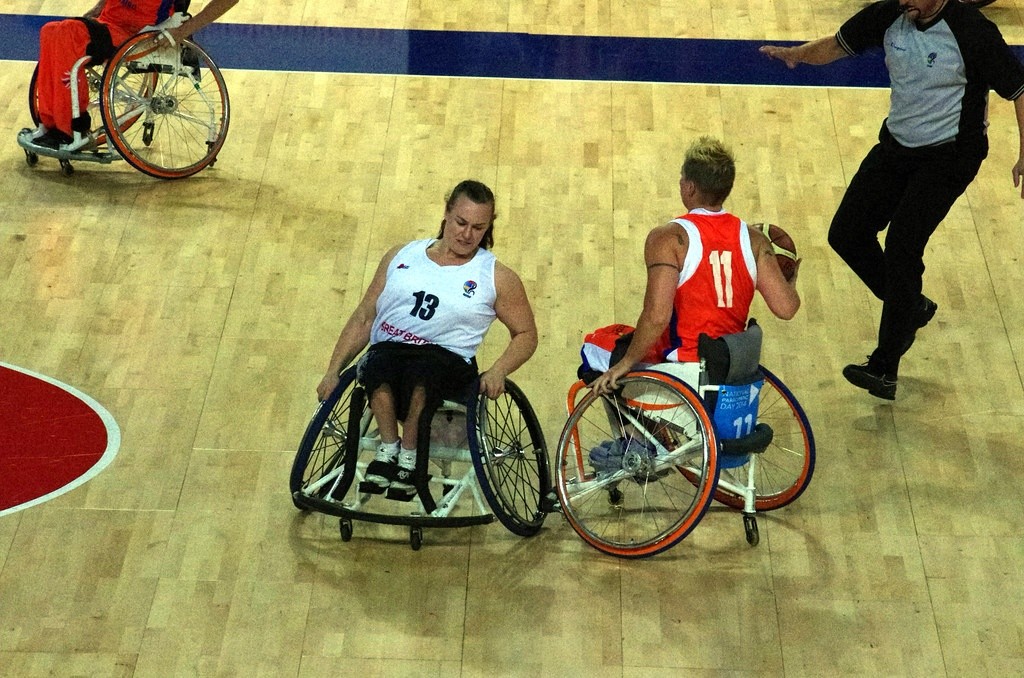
32;128;74;150
72;111;92;132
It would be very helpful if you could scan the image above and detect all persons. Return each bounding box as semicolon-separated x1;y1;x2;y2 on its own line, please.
758;0;1024;400
580;136;797;450
316;179;538;501
32;1;192;148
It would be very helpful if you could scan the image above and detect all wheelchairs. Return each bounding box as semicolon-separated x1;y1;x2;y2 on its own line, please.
17;13;231;180
289;356;550;549
544;319;815;560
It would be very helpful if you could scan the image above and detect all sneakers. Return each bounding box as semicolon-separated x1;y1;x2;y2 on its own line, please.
843;355;897;400
600;439;669;475
389;452;418;495
365;443;399;487
588;446;659;482
900;296;937;355
